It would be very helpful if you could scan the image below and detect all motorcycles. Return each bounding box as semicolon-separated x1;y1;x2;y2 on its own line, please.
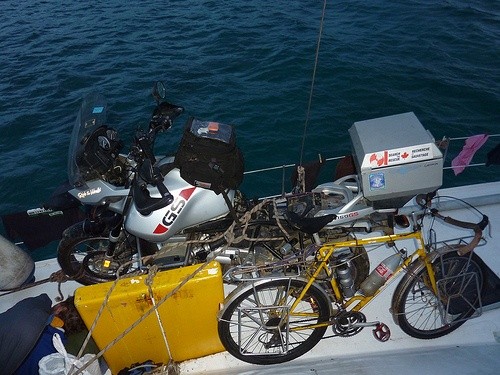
56;80;443;287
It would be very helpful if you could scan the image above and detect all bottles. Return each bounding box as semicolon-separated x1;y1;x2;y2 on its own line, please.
359;249;409;297
334;262;357;297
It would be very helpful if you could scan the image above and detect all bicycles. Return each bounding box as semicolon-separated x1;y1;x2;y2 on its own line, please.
216;196;492;366
